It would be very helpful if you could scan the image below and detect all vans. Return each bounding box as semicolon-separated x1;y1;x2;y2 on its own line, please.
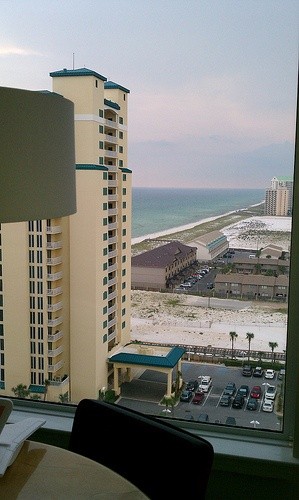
278;369;285;380
197;413;209;422
198;376;213;393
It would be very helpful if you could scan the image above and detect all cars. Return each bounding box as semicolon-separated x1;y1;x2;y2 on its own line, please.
246;398;258;411
250;386;262;399
225;417;236;425
253;366;264;377
265;369;276;379
213;419;221;423
183;414;194;420
265;385;277;400
232;394;245;409
192;392;204;405
219;395;231;407
262;399;275;412
237;384;249;397
180;390;192;402
175;264;216;291
214;249;236;263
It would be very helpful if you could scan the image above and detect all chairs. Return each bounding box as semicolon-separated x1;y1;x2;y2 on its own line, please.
67;399;214;500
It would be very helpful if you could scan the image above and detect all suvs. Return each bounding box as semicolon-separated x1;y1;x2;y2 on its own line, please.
187;378;198;392
224;383;236;396
242;364;253;377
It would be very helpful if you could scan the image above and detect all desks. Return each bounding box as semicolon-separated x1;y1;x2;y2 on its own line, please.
1;440;151;500
